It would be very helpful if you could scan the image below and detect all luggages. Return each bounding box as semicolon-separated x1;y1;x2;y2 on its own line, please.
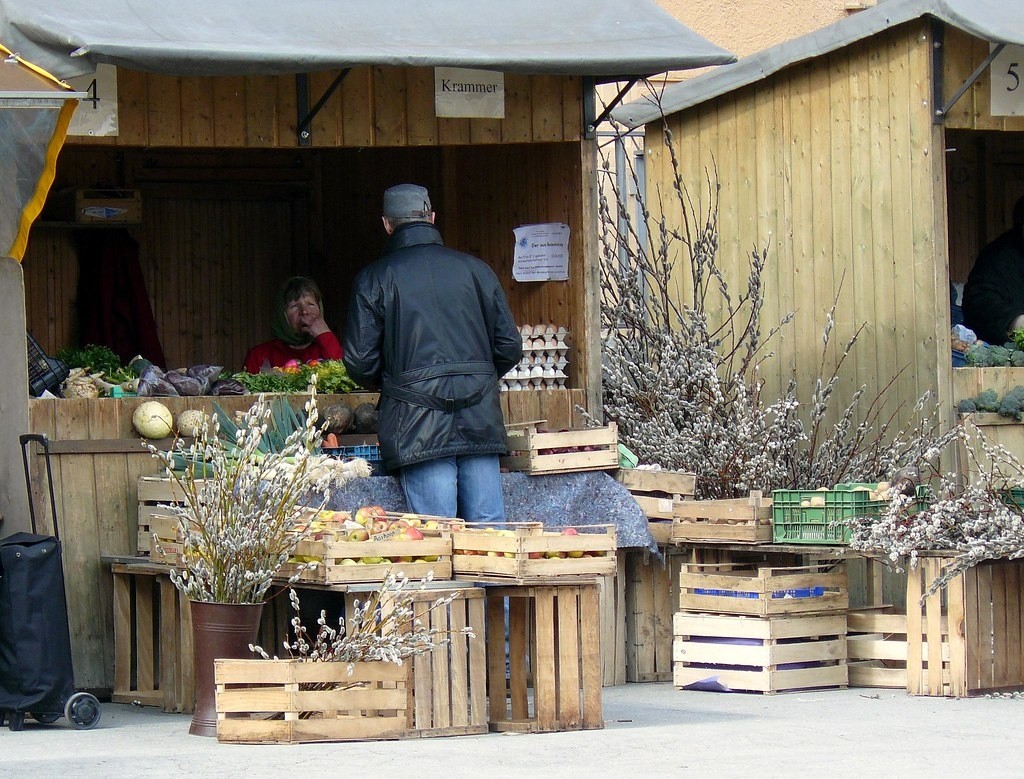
0;433;102;731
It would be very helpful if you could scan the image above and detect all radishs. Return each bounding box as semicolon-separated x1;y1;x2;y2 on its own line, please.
92;378;142;393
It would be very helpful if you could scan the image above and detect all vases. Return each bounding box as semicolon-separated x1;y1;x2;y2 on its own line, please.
188;599;264;737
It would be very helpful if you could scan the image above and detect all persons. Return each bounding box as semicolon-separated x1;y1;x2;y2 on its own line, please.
958;192;1024;344
344;182;523;696
242;275;343;374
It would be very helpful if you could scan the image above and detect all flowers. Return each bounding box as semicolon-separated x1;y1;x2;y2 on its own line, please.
572;72;1024;607
248;566;476;720
140;373;373;604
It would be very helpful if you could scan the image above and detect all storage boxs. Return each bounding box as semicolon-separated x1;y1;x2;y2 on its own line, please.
26;327;1024;746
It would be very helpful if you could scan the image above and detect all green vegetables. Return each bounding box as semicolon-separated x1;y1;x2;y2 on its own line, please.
222;360;359;395
48;342;138;382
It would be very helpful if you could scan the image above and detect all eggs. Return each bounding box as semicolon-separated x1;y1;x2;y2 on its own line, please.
514;324;561;390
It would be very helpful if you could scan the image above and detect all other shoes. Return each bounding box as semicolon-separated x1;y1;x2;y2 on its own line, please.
505;679;511;698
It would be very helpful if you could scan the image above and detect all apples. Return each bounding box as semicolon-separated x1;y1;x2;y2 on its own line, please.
274;507;608;567
800;481;908;509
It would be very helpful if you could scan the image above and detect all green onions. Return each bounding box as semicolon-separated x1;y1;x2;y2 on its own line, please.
157;392;324;480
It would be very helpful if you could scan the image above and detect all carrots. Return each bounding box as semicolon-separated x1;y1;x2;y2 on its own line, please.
318;433;338;447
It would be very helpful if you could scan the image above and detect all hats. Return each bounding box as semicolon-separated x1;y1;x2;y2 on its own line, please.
382;183;432;218
